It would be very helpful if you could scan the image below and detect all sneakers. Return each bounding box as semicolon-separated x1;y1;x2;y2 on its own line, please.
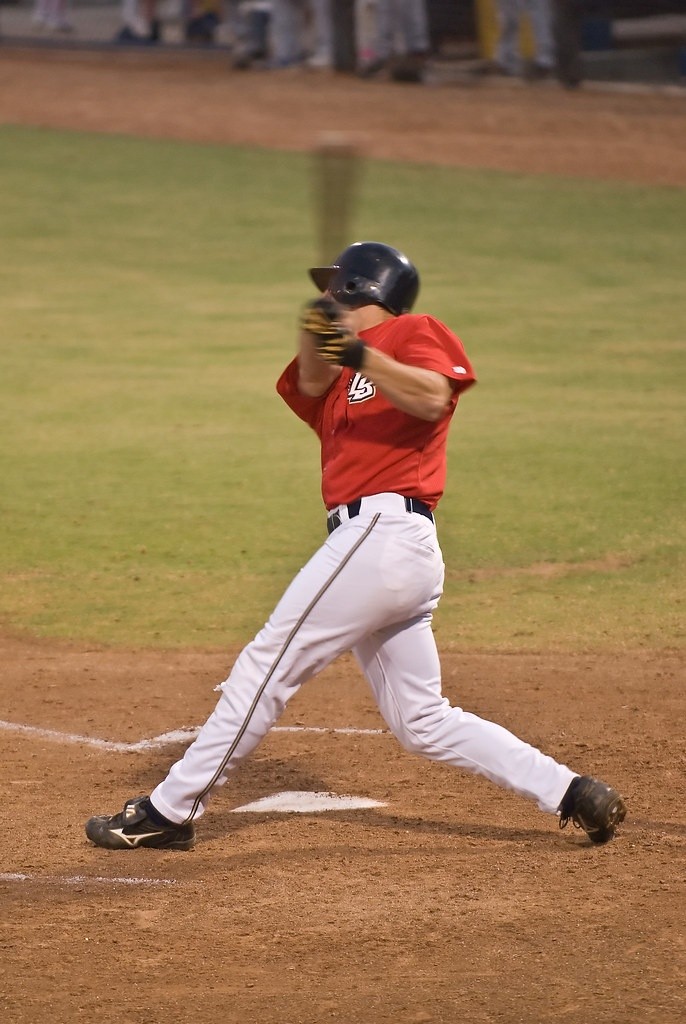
570;776;628;845
85;795;196;851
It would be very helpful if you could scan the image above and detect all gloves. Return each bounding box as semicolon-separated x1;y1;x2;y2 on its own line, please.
302;300;365;371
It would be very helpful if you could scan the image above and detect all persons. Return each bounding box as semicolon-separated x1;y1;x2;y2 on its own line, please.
28;0;627;84
85;240;627;851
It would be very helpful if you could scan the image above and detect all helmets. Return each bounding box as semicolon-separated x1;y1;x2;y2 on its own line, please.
308;241;420;317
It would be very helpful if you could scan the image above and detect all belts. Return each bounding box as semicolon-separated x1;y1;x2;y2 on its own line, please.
328;498;434;535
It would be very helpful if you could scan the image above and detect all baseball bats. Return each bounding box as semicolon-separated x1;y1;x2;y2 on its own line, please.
306;134;365;267
